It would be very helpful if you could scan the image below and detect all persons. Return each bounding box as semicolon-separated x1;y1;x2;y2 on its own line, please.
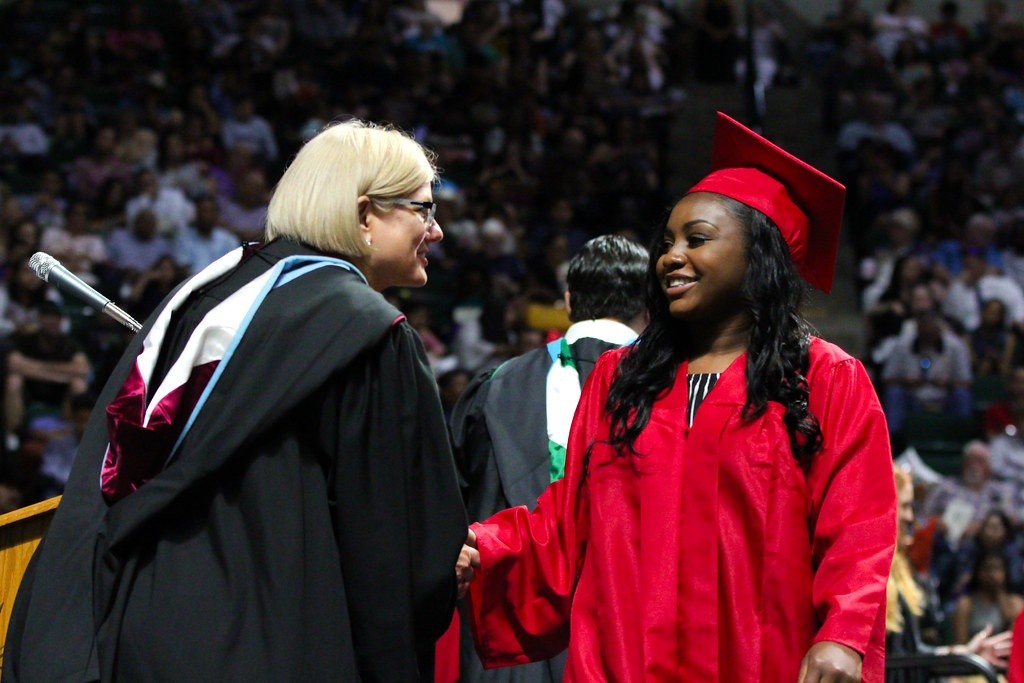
0;120;465;683
463;111;898;683
0;0;1024;683
447;234;654;683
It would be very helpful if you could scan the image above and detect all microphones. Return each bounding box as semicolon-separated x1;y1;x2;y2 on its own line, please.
27;251;143;333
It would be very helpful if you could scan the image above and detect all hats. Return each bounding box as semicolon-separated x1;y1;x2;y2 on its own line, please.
685;110;846;295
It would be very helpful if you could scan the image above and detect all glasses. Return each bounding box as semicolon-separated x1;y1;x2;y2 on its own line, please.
368;193;438;224
